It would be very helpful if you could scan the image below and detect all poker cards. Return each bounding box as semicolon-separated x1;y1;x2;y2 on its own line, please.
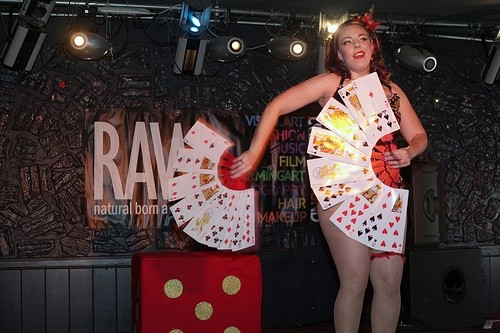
307;71;410;254
168;121;256;252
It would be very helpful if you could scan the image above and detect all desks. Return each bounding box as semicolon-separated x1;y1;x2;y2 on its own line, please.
132;251;262;333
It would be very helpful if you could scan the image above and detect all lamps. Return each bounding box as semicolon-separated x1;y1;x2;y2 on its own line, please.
66;32;111;61
227;37;246;57
392;45;437;74
265;35;308;62
480;32;500;87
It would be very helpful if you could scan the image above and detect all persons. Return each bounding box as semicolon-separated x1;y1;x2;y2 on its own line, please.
229;15;429;333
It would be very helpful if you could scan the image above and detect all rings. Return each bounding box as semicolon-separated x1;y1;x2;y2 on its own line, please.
240;160;244;164
